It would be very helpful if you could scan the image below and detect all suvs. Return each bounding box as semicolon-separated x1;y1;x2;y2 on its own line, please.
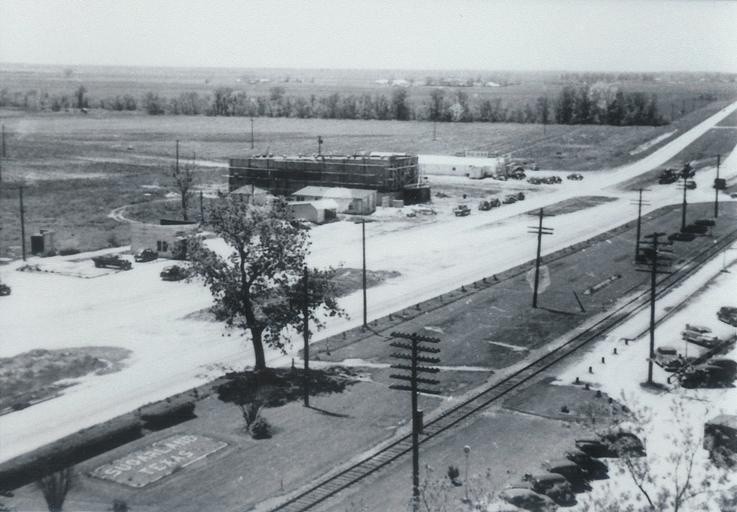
655;347;682;370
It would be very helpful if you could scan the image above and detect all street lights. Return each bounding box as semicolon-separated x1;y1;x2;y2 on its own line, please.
174;136;179;173
464;446;471;499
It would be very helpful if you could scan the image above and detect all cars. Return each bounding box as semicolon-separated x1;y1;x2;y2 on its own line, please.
455;204;470;216
527;173;583;184
720;307;737;326
659;164;694;182
479;191;524;210
685;325;718;348
